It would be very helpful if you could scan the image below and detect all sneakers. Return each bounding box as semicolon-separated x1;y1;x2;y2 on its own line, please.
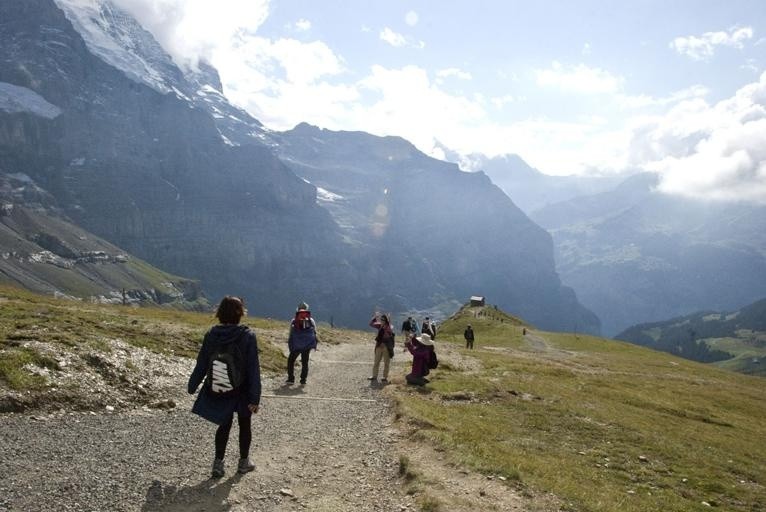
212;458;225;477
285;378;295;383
238;458;255;473
301;378;306;384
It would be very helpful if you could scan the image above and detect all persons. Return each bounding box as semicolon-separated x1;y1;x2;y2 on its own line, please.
522;328;525;336
472;310;503;323
401;315;436;343
186;295;260;476
284;302;317;386
405;333;438;386
367;312;393;381
329;316;335;329
462;325;474;350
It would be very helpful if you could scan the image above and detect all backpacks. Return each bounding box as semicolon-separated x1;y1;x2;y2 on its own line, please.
204;329;250;396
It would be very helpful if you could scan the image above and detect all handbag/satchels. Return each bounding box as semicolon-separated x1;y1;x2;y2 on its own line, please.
429;352;438;368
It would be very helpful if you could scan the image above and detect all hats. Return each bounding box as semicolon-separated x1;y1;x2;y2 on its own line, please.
416;333;434;345
